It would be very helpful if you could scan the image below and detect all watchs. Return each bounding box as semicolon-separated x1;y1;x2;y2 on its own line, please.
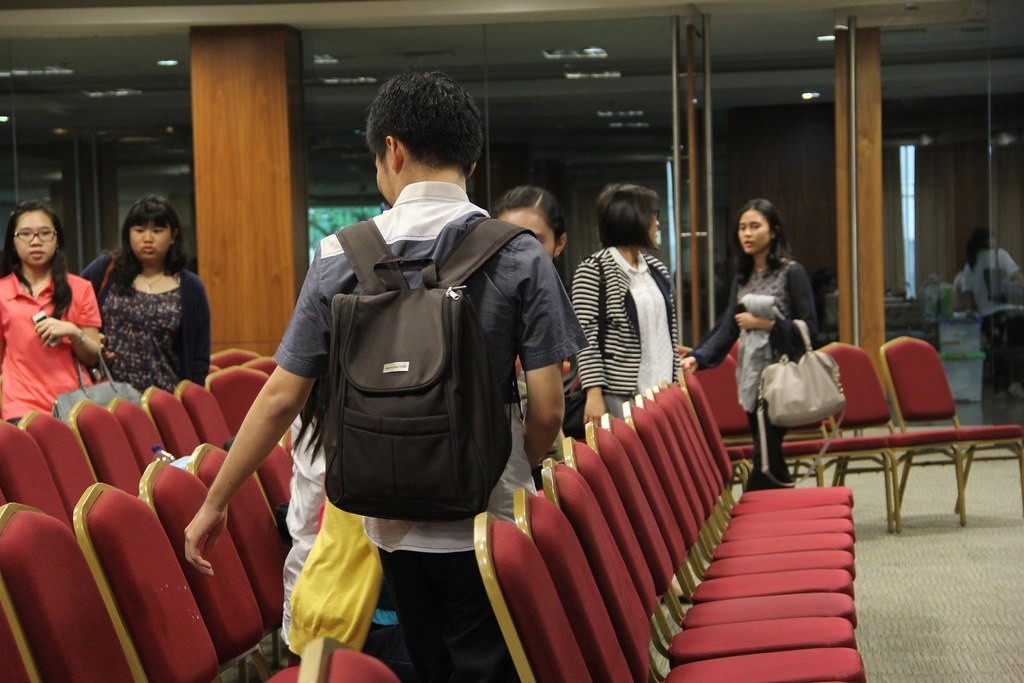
68;329;85;346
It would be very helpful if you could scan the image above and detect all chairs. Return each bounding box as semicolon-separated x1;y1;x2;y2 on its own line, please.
0;339;1024;683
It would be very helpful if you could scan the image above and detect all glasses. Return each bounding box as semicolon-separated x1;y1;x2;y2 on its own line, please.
15;228;57;242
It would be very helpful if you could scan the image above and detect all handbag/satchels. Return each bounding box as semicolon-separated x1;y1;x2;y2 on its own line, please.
983;248;1009;304
757;319;847;486
51;345;143;424
562;390;587;439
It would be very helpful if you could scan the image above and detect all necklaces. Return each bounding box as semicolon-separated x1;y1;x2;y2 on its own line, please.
754;267;765;273
143;270;165;294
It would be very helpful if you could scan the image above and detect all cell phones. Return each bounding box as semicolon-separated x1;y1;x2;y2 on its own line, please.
32;310;61;347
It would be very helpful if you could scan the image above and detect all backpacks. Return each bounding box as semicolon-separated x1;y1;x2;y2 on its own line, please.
323;218;538;523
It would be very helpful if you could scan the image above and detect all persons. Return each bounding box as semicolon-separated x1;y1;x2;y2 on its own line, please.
78;195;210;396
183;73;573;683
680;199;819;492
952;226;1024;399
284;185;684;683
0;199;103;430
